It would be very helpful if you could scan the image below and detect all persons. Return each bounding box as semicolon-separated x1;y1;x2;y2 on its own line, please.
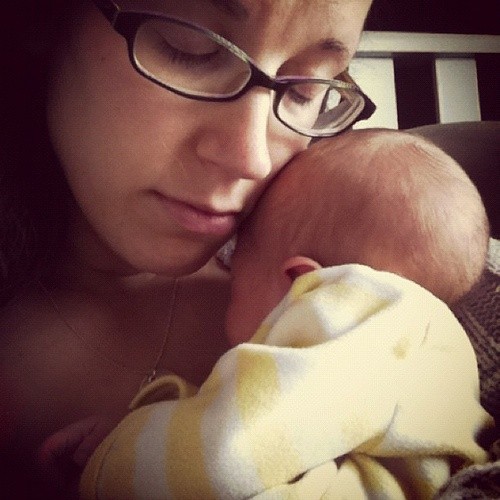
38;129;495;499
0;0;500;498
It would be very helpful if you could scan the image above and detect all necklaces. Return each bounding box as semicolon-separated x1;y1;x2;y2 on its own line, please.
36;275;180;390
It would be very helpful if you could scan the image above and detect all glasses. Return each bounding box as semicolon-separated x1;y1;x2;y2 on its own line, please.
99;0;375;138
